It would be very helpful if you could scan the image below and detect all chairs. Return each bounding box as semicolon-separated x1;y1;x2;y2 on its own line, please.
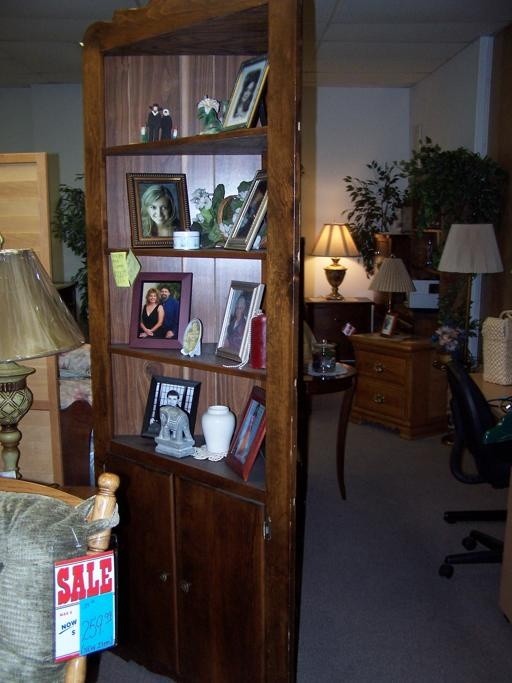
56;342;95;487
1;471;126;683
439;360;512;578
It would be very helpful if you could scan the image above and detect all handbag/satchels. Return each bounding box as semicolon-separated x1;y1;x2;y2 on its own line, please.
486;395;512;489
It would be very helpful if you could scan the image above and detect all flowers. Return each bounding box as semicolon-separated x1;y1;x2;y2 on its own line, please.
196;94;222;124
431;323;470;363
189;179;267;250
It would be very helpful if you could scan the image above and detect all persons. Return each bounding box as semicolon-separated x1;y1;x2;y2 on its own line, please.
159;107;173;140
225;296;252;357
237;402;262;460
136;288;165;336
157;284;181;340
139;185;184;235
234;73;256;116
152;389;186;432
142;102;164;142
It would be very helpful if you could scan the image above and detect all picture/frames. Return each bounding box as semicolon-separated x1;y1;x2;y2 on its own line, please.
224;168;268;252
225;385;267;480
140;376;201;443
214;279;265;363
127;270;193;350
380;312;398;338
126;172;192;249
221;54;269;131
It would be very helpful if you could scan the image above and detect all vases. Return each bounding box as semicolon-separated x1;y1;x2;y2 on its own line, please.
201;117;219;134
202;403;235;454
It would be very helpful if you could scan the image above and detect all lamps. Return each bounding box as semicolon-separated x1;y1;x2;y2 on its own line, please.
368;256;417;333
0;247;87;491
309;223;361;301
438;222;505;369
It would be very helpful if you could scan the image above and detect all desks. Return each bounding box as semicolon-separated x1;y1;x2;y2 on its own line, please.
348;330;449;442
302;363;358;500
469;370;512;418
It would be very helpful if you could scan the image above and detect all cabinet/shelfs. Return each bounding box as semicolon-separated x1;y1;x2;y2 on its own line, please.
0;152;66;488
52;281;77;321
78;1;306;653
